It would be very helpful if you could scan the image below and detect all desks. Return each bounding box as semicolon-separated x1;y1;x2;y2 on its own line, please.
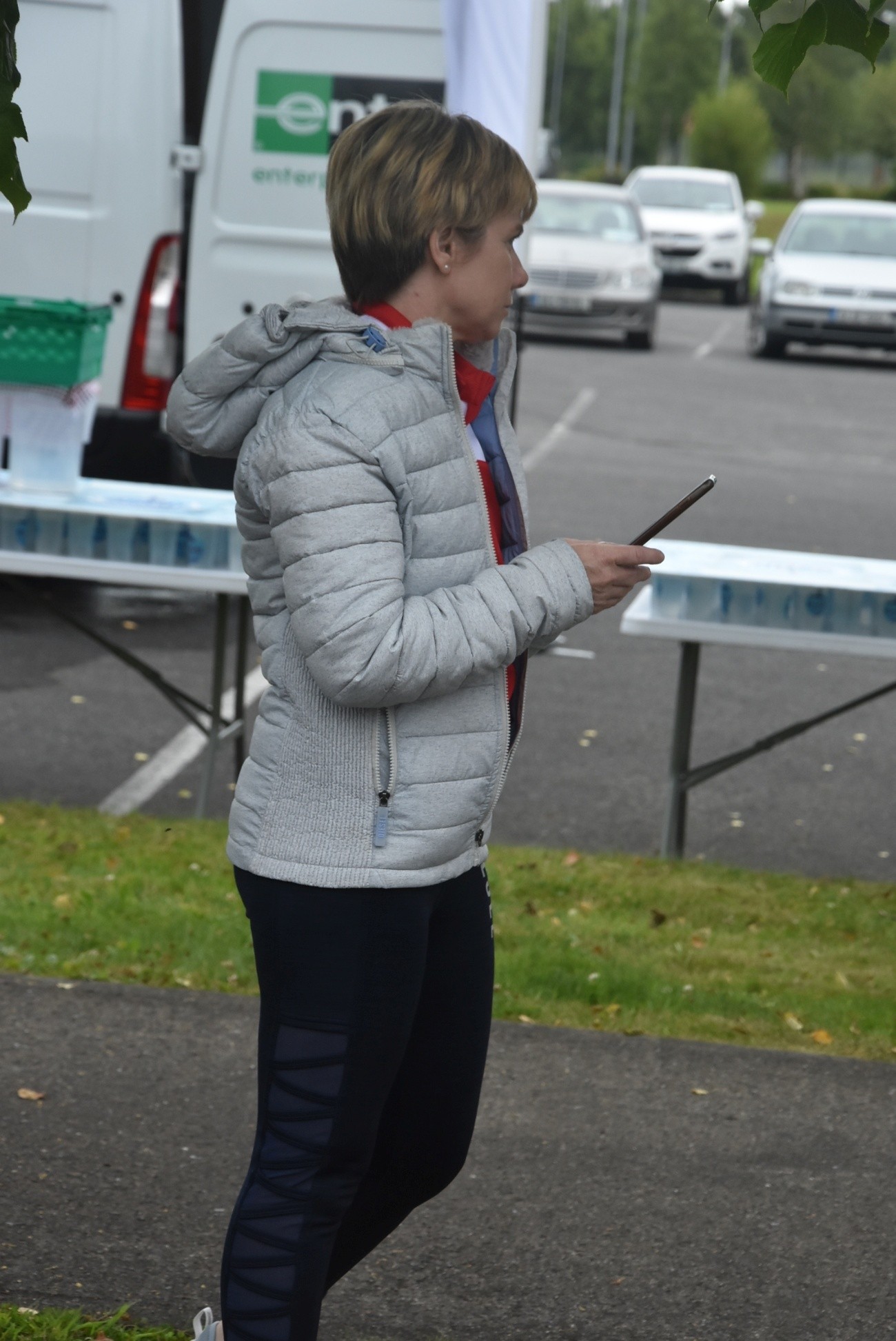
620;584;896;861
0;525;250;819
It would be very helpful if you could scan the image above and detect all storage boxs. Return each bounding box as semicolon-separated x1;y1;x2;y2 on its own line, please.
0;292;113;384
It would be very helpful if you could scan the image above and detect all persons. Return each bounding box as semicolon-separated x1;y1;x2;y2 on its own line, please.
166;100;665;1341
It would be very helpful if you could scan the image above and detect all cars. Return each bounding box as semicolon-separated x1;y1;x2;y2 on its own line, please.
744;198;896;358
513;177;666;345
619;165;769;304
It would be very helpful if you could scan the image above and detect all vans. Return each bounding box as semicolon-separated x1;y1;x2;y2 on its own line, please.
1;0;553;617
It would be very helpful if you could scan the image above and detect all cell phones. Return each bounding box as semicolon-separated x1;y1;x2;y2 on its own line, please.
627;475;719;546
